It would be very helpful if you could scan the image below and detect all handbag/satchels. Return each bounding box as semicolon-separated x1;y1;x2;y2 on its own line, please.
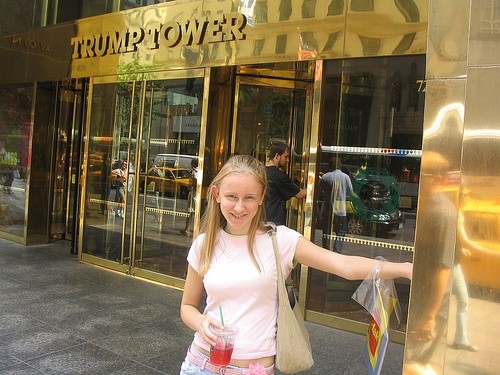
275;223;316;372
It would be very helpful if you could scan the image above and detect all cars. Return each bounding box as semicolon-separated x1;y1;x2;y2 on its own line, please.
139;166;194;199
313;171;404;236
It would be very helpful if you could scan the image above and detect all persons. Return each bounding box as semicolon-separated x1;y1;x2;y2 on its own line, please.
180;158;198;236
263;142;307;226
404;151;500;375
115;161;127;218
318;157;353;254
180;155;414;375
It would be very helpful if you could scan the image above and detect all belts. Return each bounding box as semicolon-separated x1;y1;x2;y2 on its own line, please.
187;347;275;375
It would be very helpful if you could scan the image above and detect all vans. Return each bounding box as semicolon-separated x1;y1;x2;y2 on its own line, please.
152;153;198;179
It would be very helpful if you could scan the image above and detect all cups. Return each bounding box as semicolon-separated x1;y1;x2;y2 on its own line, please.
209;323;240;367
